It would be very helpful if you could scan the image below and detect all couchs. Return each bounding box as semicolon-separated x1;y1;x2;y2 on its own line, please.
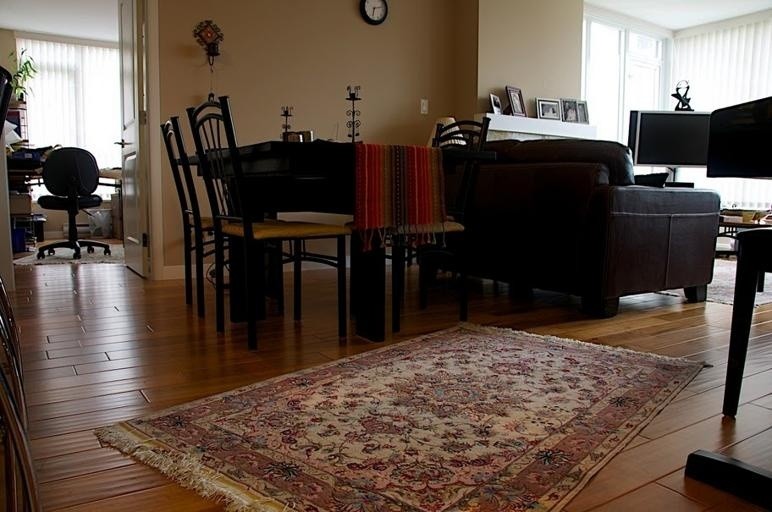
418;139;721;319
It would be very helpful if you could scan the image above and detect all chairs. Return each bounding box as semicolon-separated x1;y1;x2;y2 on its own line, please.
36;148;112;260
158;117;285;323
186;96;353;349
344;117;491;333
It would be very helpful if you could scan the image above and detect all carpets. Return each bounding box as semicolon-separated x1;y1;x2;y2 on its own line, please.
656;258;771;307
12;242;128;267
92;324;714;511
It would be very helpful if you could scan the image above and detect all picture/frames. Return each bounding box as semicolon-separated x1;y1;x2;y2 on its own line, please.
579;102;589;124
485;93;503;115
503;85;527;117
562;100;579;123
535;98;562;122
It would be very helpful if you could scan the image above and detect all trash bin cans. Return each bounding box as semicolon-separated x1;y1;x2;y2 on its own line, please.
13;227;26;252
87;208;112;238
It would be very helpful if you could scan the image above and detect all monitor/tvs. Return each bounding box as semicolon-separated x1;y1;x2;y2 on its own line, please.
633;112;710;167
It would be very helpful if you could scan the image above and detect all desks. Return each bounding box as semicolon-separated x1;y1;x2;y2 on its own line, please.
685;96;772;512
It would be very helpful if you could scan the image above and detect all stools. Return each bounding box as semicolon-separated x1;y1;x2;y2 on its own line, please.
722;230;771;419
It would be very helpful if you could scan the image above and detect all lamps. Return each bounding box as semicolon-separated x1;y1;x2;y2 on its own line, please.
194;21;224;65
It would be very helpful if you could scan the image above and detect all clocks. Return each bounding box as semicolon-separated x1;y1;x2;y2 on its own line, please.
359;0;389;25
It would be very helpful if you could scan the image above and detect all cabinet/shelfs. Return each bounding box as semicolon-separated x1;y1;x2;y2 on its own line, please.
4;108;47;254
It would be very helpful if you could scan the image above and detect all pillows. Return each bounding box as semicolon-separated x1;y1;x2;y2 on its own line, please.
634;172;669;188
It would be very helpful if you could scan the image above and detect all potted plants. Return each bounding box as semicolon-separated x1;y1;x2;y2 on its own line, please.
6;48;38;108
